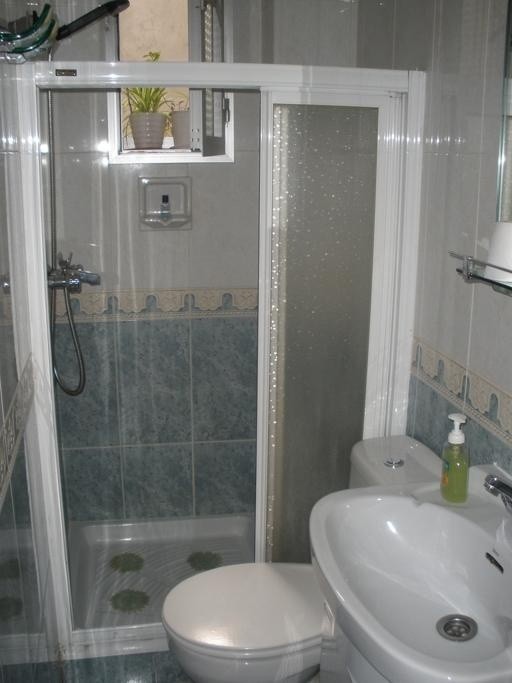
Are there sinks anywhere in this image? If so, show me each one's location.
[310,465,512,682]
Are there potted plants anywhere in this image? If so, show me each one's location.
[119,53,193,149]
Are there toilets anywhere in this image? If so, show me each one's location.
[161,435,448,683]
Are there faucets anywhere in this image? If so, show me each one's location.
[484,475,512,510]
